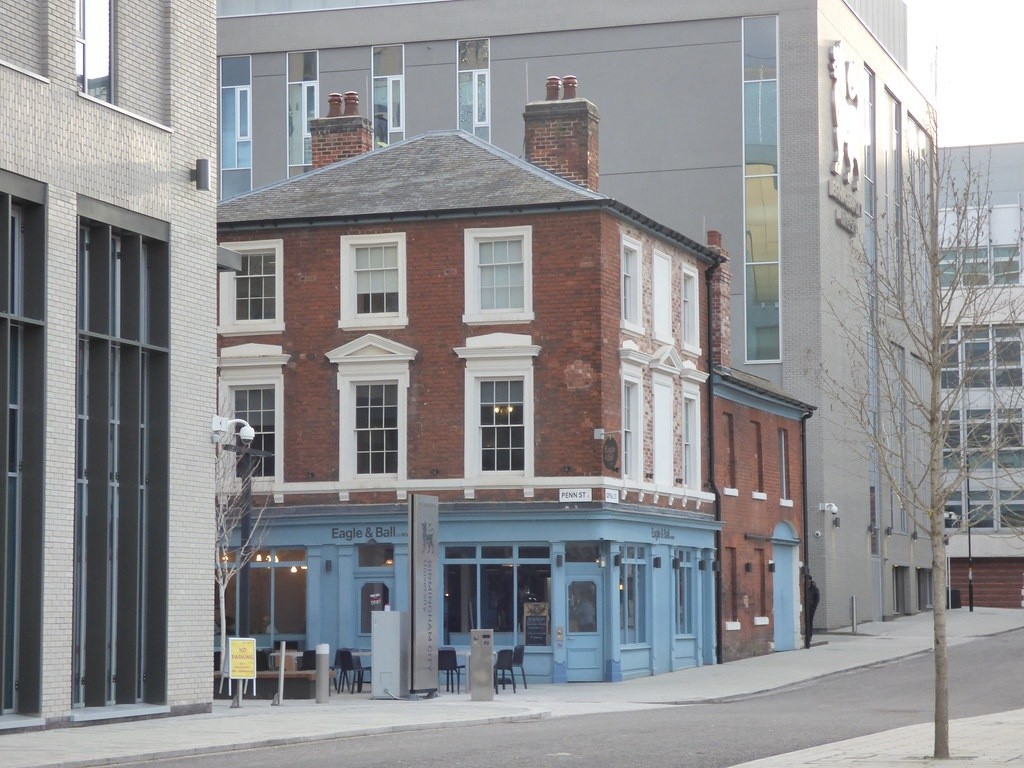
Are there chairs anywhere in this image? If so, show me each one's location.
[330,648,349,691]
[337,652,361,694]
[502,645,530,689]
[436,647,466,694]
[350,656,371,692]
[495,649,518,694]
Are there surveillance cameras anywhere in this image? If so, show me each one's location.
[831,506,839,514]
[240,425,255,445]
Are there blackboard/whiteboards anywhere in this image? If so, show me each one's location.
[525,615,547,646]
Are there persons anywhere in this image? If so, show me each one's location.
[808,574,822,642]
